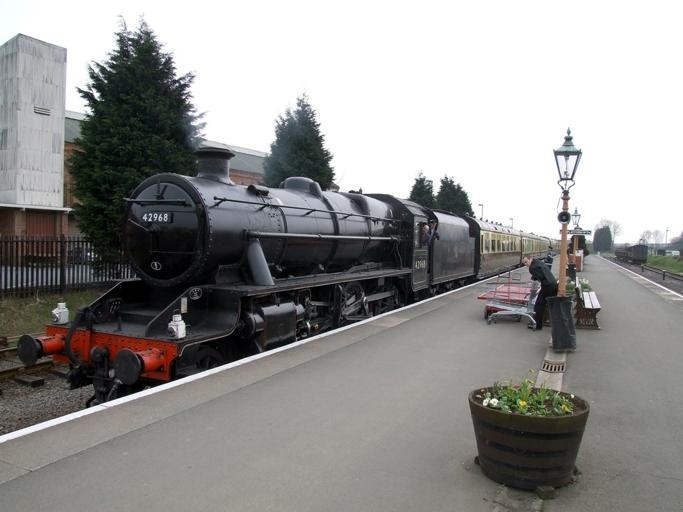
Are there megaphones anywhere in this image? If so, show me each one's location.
[557,211,571,224]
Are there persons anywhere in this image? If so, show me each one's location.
[522,254,558,330]
[539,252,553,271]
[421,224,431,247]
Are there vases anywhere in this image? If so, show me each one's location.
[468,387,590,491]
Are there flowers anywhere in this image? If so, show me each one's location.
[475,368,575,416]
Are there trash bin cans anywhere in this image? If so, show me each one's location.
[546,296,577,352]
[566,264,576,285]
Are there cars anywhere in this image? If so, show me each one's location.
[67,245,98,265]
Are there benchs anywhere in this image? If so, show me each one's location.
[574,276,601,329]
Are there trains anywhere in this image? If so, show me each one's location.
[15,141,560,406]
[615,243,649,266]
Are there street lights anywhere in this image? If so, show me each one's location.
[507,217,515,227]
[548,128,583,295]
[569,206,583,266]
[476,203,485,216]
[665,229,670,249]
